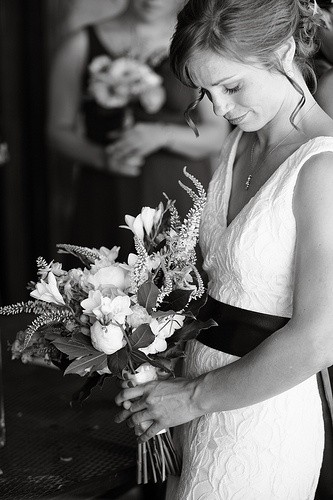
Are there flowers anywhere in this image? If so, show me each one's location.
[0,165,219,485]
[87,55,166,177]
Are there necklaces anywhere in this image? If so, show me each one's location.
[244,101,317,189]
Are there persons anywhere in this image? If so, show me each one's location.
[36,1,234,275]
[112,0,332,500]
